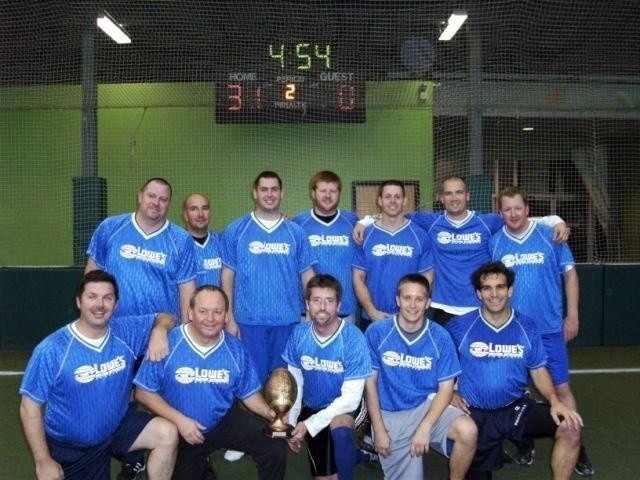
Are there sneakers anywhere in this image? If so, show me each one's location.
[117,458,146,480]
[572,444,595,476]
[358,433,382,468]
[513,445,536,466]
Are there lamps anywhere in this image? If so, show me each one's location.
[433,9,468,41]
[97,9,132,44]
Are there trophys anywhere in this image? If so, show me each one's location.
[262,366,298,441]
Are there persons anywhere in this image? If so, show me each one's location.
[179,191,226,293]
[285,170,364,332]
[486,184,597,478]
[440,260,586,480]
[361,273,480,479]
[126,283,303,480]
[345,178,437,330]
[214,170,319,462]
[350,175,572,331]
[11,268,181,479]
[279,273,379,479]
[78,177,199,480]
